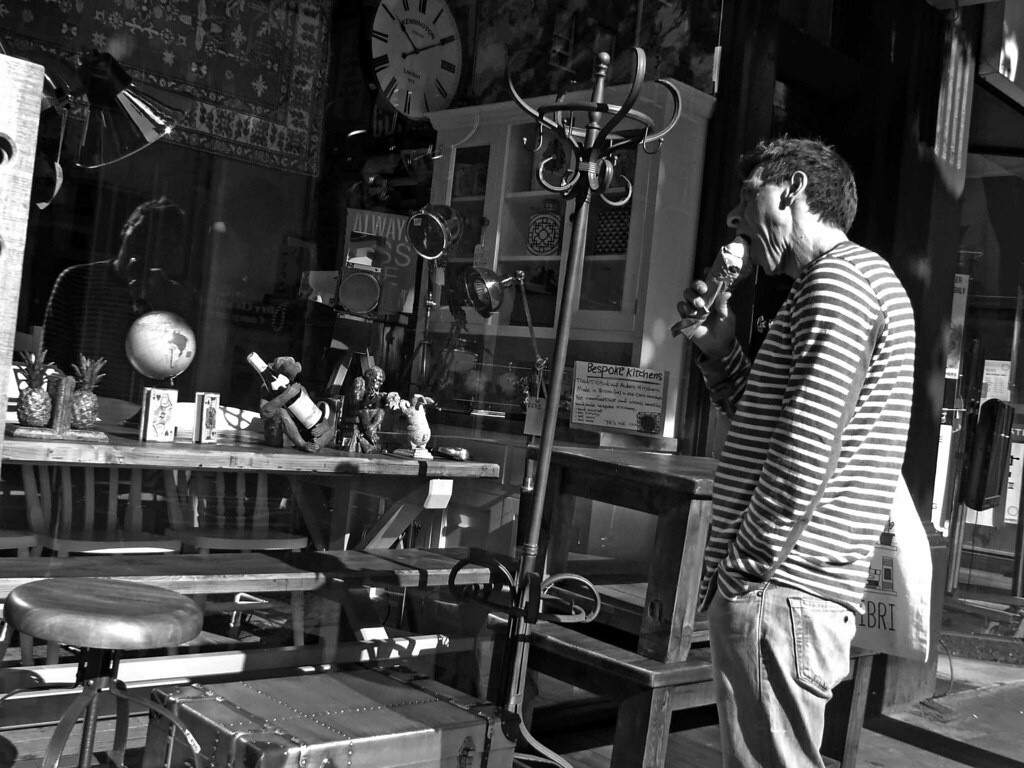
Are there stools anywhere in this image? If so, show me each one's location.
[1,576,205,768]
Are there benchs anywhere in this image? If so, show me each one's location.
[1,549,641,597]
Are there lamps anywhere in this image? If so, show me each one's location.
[405,200,547,420]
[41,45,183,169]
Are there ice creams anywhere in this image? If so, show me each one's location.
[710,236,752,297]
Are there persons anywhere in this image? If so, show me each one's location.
[355,366,388,443]
[38,196,188,407]
[677,137,916,768]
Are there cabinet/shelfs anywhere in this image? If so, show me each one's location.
[393,78,718,631]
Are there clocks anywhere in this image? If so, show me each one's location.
[361,1,466,129]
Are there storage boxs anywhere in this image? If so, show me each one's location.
[140,666,521,768]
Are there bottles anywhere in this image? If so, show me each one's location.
[246,351,324,441]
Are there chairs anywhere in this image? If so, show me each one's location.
[166,403,313,647]
[2,328,181,664]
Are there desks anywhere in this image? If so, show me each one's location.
[385,444,877,768]
[1,424,501,704]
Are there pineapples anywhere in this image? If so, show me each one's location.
[68,353,107,431]
[17,350,56,428]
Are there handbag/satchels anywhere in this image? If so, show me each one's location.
[850,471,933,663]
